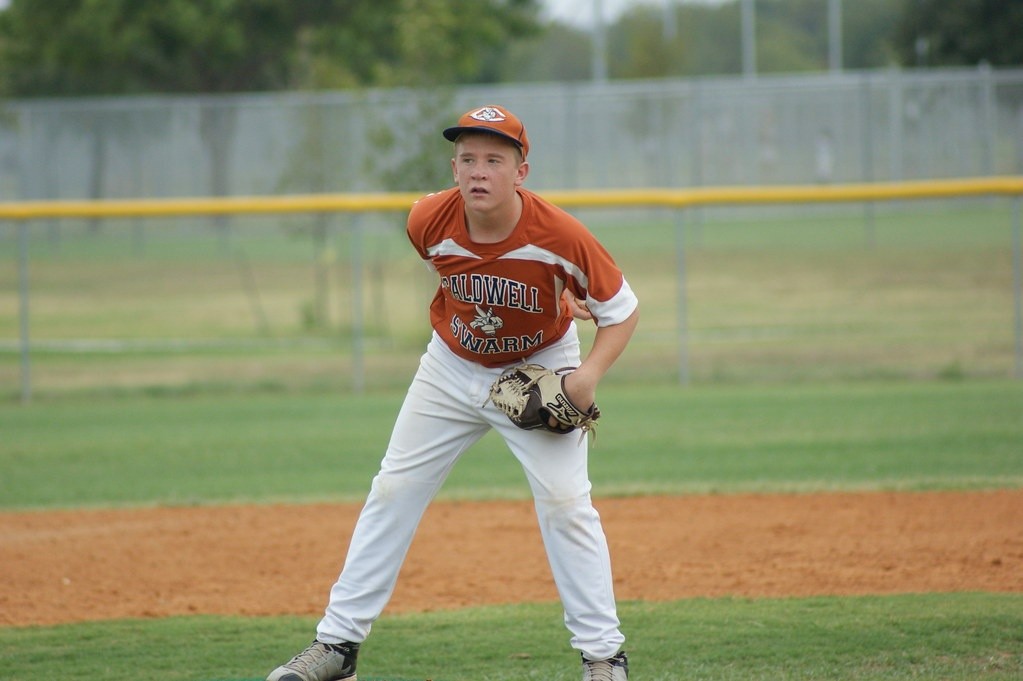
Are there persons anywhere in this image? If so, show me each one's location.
[264,106,640,680]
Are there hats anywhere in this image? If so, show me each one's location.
[442,105,529,162]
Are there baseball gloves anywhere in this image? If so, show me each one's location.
[489,364,601,436]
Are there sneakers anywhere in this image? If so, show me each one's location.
[265,638,361,681]
[581,649,628,681]
